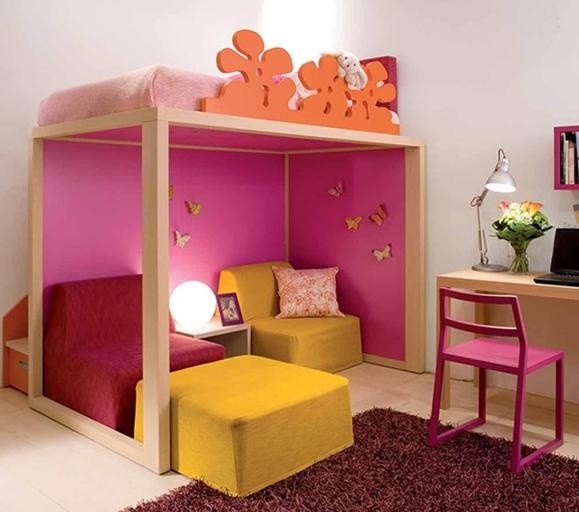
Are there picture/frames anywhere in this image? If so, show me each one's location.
[215,292,244,327]
[554,124,579,191]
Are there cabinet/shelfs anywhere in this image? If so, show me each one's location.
[5,337,28,397]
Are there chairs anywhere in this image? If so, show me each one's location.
[216,261,364,375]
[426,287,565,475]
[44,273,225,435]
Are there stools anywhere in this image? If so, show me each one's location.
[134,354,356,497]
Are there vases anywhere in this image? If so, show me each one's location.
[504,250,532,271]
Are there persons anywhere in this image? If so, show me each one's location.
[222,298,239,320]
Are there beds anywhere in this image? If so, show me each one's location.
[27,29,403,139]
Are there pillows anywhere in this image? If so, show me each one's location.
[269,263,344,318]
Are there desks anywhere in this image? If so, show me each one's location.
[436,268,579,411]
[175,315,251,355]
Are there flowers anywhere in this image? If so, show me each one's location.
[488,198,552,271]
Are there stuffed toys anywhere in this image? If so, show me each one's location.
[319,49,368,92]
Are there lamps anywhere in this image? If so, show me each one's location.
[470,146,517,272]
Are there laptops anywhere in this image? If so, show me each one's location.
[534,228,579,286]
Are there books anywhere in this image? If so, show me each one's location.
[560,128,579,184]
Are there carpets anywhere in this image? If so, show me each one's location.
[121,407,579,512]
